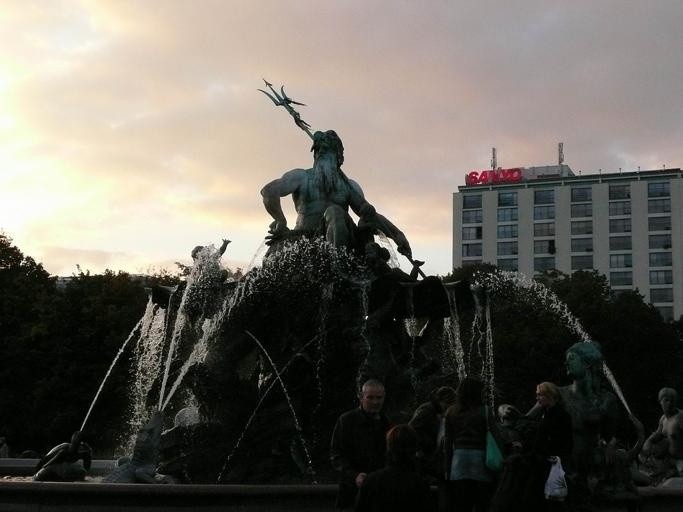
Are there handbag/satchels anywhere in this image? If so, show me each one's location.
[542,455,571,504]
[483,429,525,483]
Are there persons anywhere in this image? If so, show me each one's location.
[375,244,426,283]
[497,381,572,512]
[327,379,389,512]
[408,384,454,485]
[260,130,412,271]
[360,242,382,293]
[440,374,513,511]
[496,337,644,502]
[353,422,434,511]
[634,385,683,493]
